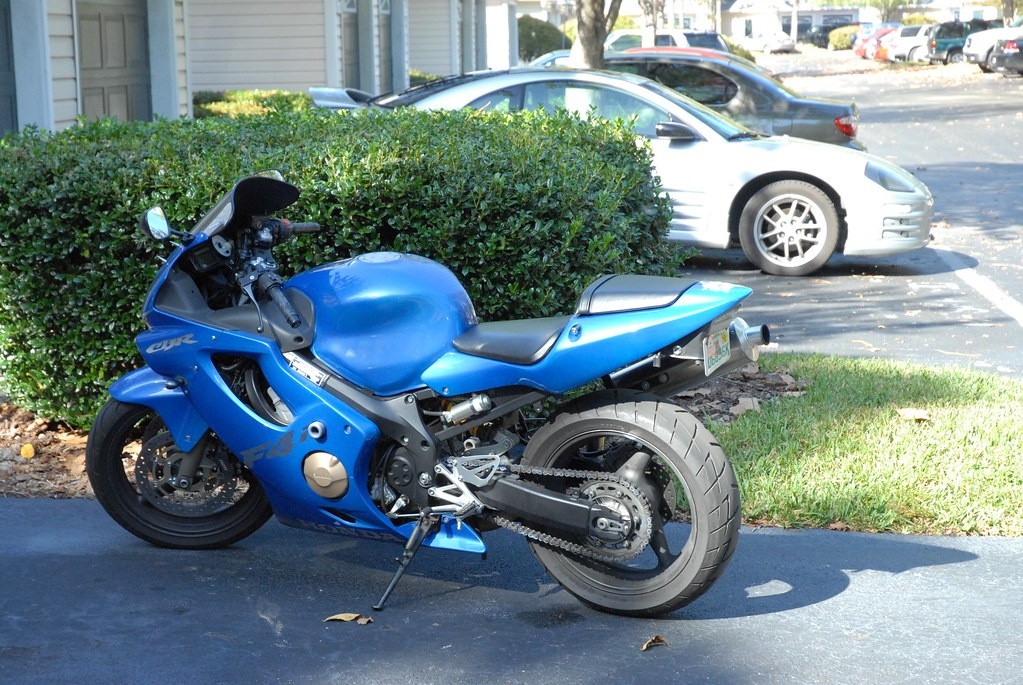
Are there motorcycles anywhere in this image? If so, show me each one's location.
[83,165,772,617]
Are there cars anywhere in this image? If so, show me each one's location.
[736,27,795,56]
[812,14,1023,74]
[525,27,870,154]
[305,66,937,277]
